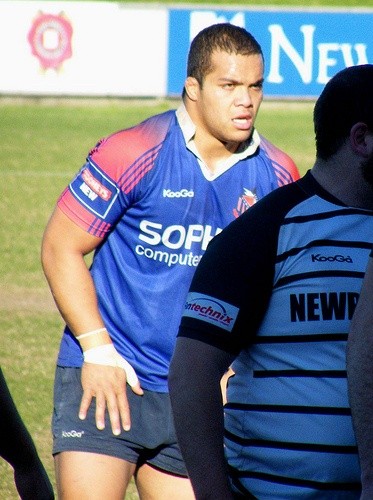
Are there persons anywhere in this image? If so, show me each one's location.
[0,370,54,500]
[344,251,373,500]
[169,64,373,500]
[40,24,302,500]
